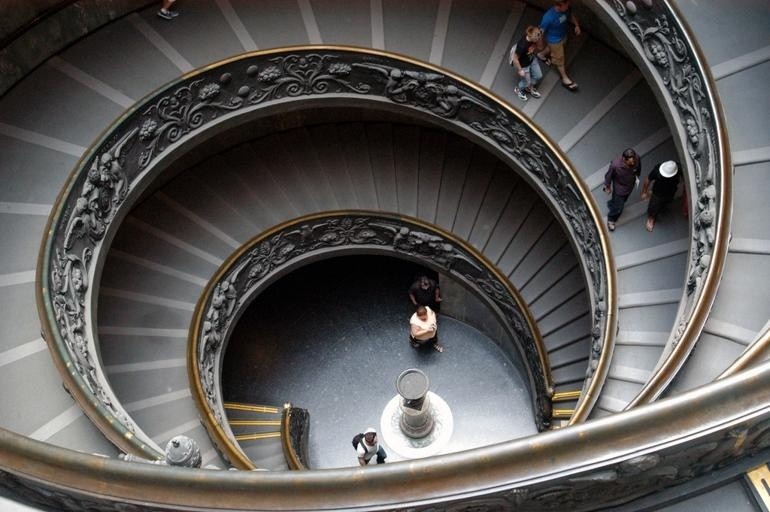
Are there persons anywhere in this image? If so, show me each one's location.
[601,147,641,232]
[507,23,544,101]
[537,1,582,93]
[409,305,443,352]
[407,274,443,311]
[641,159,683,232]
[155,0,181,21]
[351,426,387,465]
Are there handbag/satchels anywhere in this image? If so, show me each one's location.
[509,42,518,65]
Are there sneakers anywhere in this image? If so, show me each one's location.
[433,344,444,353]
[607,220,616,232]
[514,87,528,101]
[646,219,655,232]
[409,340,420,348]
[530,88,541,98]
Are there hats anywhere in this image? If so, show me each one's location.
[659,160,679,178]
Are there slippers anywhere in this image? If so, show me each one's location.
[562,79,578,90]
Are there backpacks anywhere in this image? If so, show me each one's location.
[352,433,364,451]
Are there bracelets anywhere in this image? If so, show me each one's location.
[644,191,647,193]
[574,25,580,27]
